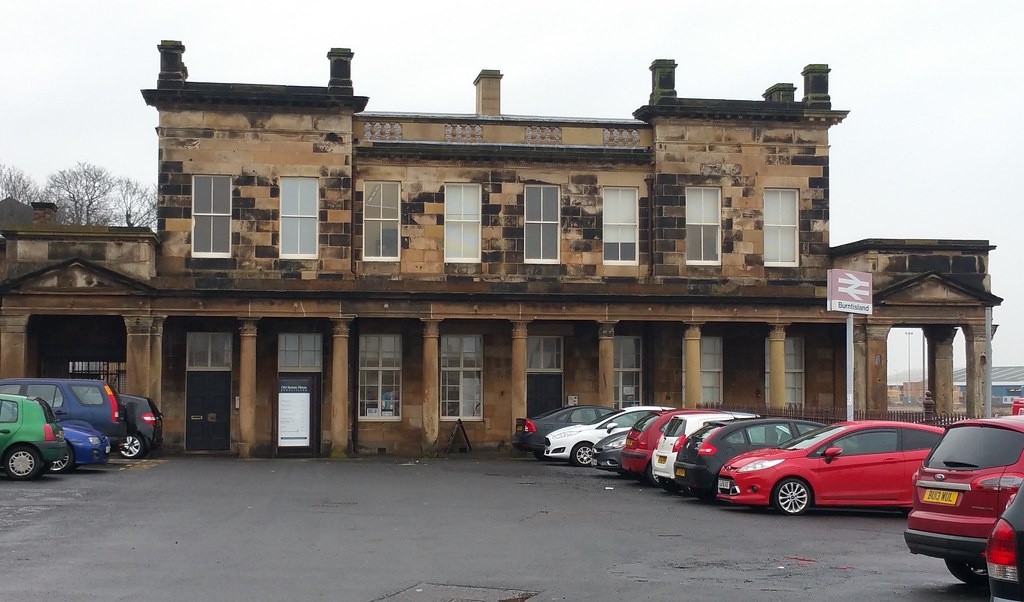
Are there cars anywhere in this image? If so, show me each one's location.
[716,417,947,516]
[512,404,619,463]
[619,408,719,488]
[590,427,631,477]
[0,394,68,481]
[117,393,163,460]
[650,411,795,500]
[542,402,672,467]
[58,422,111,472]
[905,414,1024,588]
[674,415,827,503]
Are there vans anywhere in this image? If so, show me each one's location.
[1,377,128,449]
